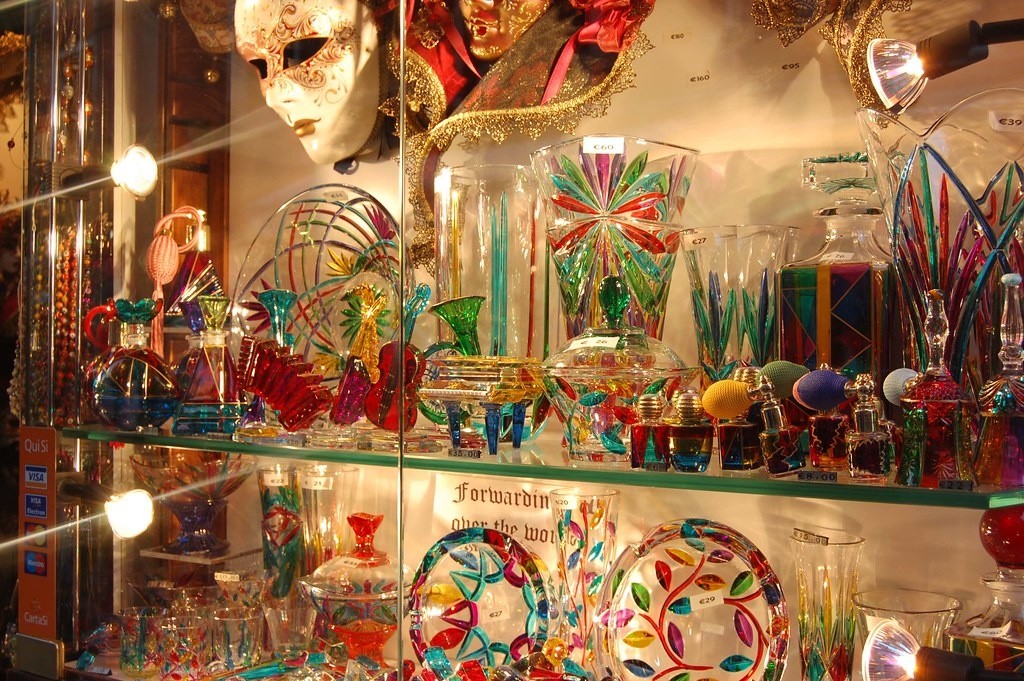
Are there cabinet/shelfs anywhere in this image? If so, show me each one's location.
[60,418,1024,681]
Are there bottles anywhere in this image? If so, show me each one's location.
[78,84,1024,680]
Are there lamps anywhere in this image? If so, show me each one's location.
[866,17,1024,114]
[57,475,154,542]
[59,144,159,201]
[861,619,1024,681]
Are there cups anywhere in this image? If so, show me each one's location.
[118,596,318,681]
